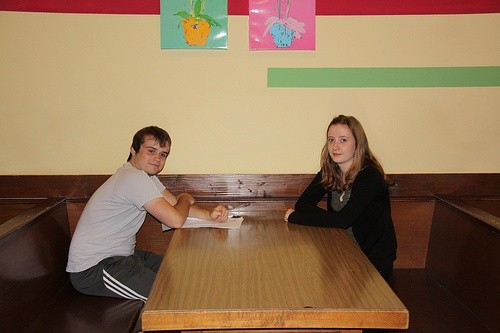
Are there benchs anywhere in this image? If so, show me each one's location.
[0,196,144,333]
[387,194,500,333]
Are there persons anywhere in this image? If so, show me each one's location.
[66,126,228,333]
[284,115,397,281]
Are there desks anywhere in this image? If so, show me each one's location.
[139,210,410,333]
